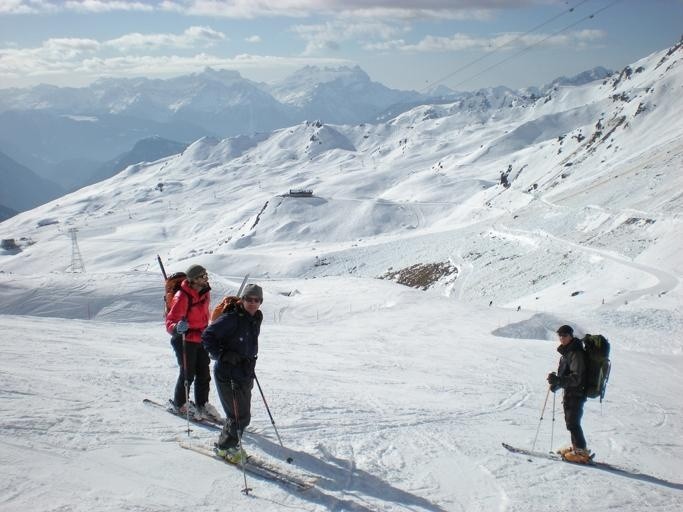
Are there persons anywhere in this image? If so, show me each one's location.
[197,283,262,464]
[546,324,589,463]
[163,263,219,424]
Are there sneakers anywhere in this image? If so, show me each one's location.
[213,445,246,465]
[167,400,201,421]
[565,450,592,464]
[197,402,225,425]
[559,445,591,454]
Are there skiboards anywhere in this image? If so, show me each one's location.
[501,442,638,479]
[141,398,261,439]
[175,435,318,491]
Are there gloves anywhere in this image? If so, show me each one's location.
[177,321,189,334]
[221,351,240,365]
[548,374,557,383]
[551,384,558,392]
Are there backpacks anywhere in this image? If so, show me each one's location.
[164,272,186,312]
[208,295,242,328]
[582,333,611,399]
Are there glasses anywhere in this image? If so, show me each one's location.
[197,273,208,279]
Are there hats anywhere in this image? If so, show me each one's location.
[186,265,205,281]
[242,284,263,300]
[556,325,573,334]
